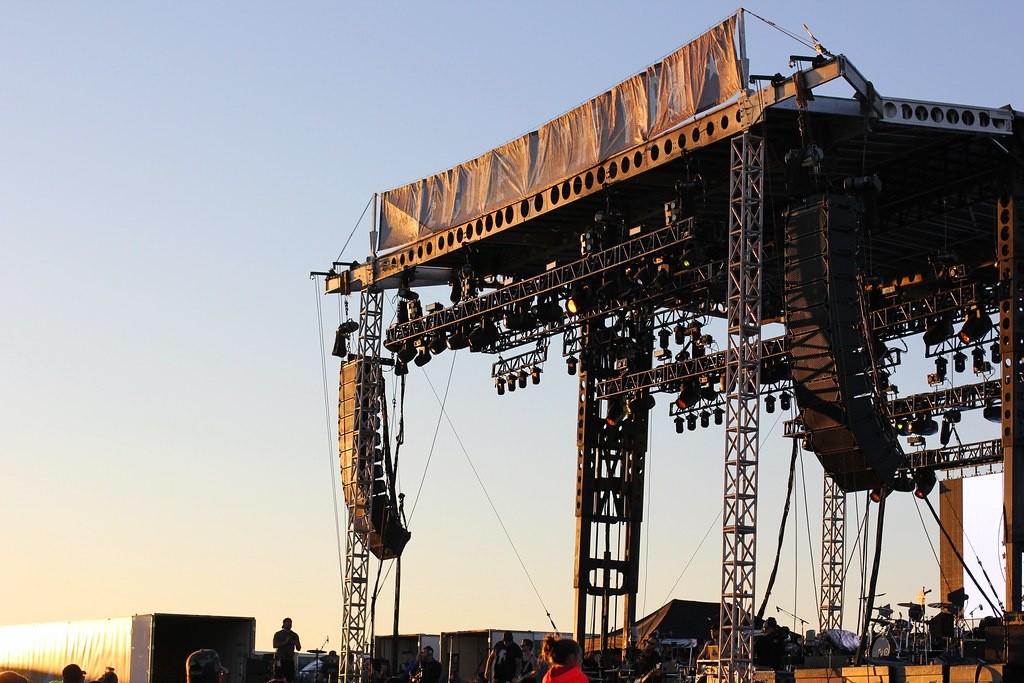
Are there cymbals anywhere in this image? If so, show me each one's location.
[872,607,894,613]
[897,603,921,608]
[870,618,892,627]
[927,602,953,609]
[307,650,327,653]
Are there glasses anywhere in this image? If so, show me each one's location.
[216,671,225,679]
[79,676,85,681]
[520,644,528,648]
[647,641,656,648]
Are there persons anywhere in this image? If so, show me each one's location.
[0,616,784,683]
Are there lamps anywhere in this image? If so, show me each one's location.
[381,158,1004,504]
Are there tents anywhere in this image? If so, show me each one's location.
[578,598,803,667]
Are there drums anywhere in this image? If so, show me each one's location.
[869,634,898,660]
[896,619,913,632]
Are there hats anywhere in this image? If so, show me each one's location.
[710,623,719,630]
[402,649,413,655]
[502,631,513,642]
[186,649,229,674]
[63,664,86,678]
[282,618,292,628]
